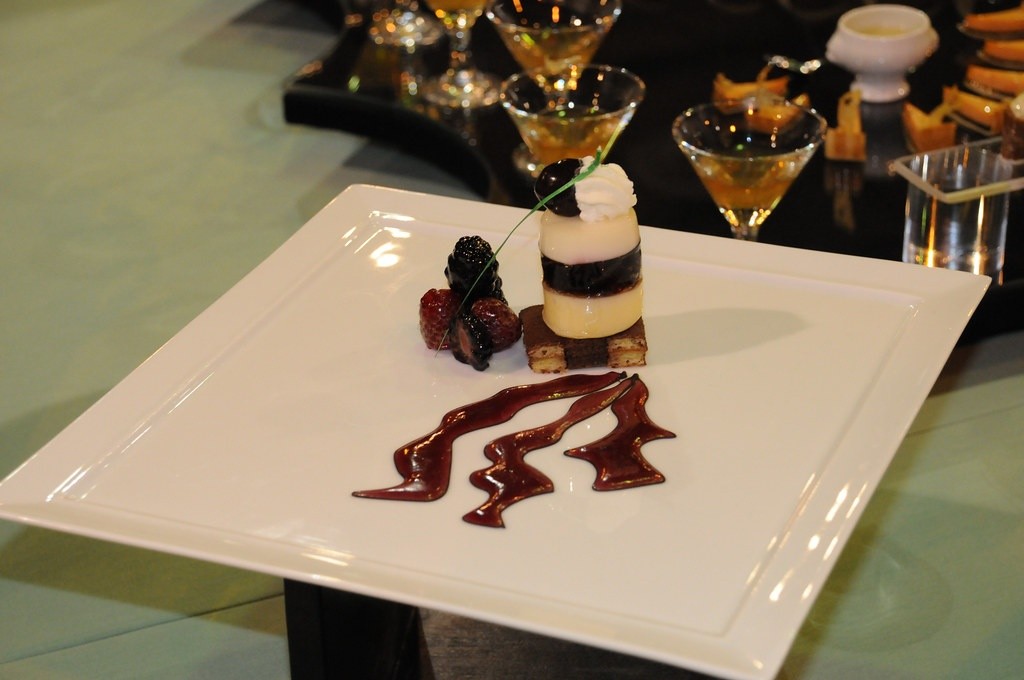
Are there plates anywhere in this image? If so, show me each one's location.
[0,181,990,680]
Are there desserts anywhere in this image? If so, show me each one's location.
[518,154,647,375]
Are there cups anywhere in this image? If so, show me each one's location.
[822,4,940,105]
[889,146,1020,277]
[672,96,829,243]
[364,0,646,167]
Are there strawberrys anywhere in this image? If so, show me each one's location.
[418,289,524,354]
